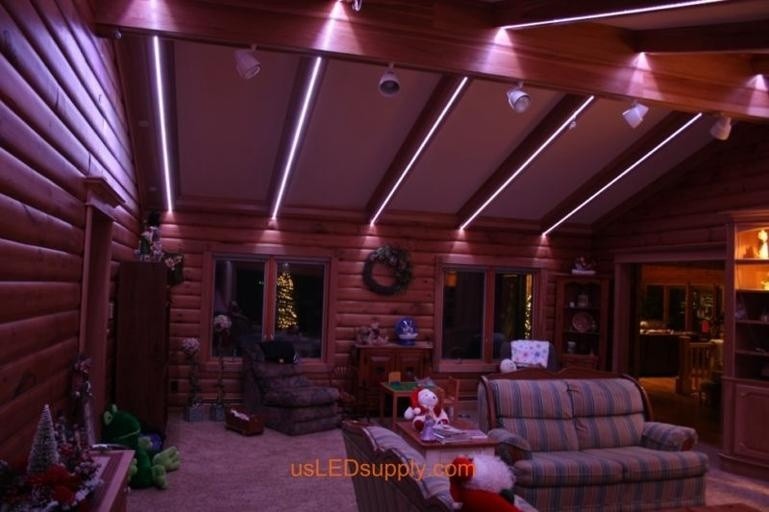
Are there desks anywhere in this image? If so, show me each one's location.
[59,448,138,512]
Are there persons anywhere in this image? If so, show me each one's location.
[403,385,451,430]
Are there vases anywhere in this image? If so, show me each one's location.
[184,404,225,426]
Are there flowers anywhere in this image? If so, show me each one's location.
[178,314,231,406]
[362,246,411,296]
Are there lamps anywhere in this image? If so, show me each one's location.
[232,48,736,142]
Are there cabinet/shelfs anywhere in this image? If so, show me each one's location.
[627,206,769,480]
[555,264,607,376]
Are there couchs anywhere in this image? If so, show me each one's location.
[340,336,709,511]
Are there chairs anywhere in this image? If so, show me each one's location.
[241,342,340,436]
